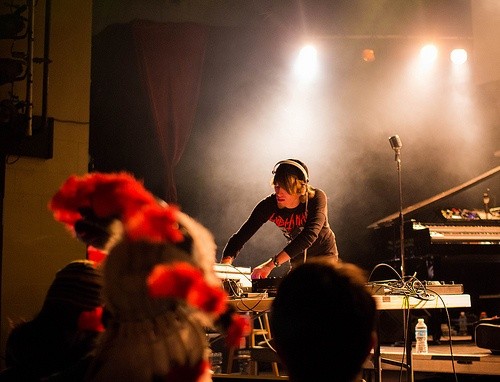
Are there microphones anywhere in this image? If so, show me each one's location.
[388,135,402,150]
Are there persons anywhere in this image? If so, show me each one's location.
[0,172,381,382]
[221,159,338,280]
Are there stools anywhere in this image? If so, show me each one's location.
[225,310,279,377]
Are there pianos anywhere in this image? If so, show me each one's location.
[364,165,500,345]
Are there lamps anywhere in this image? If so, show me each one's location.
[0,99,28,125]
[0,57,28,86]
[0,4,31,40]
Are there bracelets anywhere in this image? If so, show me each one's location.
[272,256,280,268]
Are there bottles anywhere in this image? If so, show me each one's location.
[459,312,467,335]
[480,311,487,318]
[414,318,427,353]
